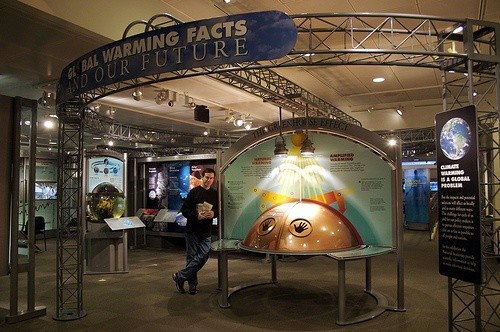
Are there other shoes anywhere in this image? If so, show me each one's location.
[189,285,197,294]
[172,273,186,294]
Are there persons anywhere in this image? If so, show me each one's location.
[172,168,218,295]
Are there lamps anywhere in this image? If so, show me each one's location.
[132,87,253,135]
[396,106,408,116]
[42,91,54,109]
[300,104,315,154]
[273,106,288,156]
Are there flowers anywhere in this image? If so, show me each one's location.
[92,196,115,223]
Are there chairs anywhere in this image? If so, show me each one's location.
[20,215,46,251]
